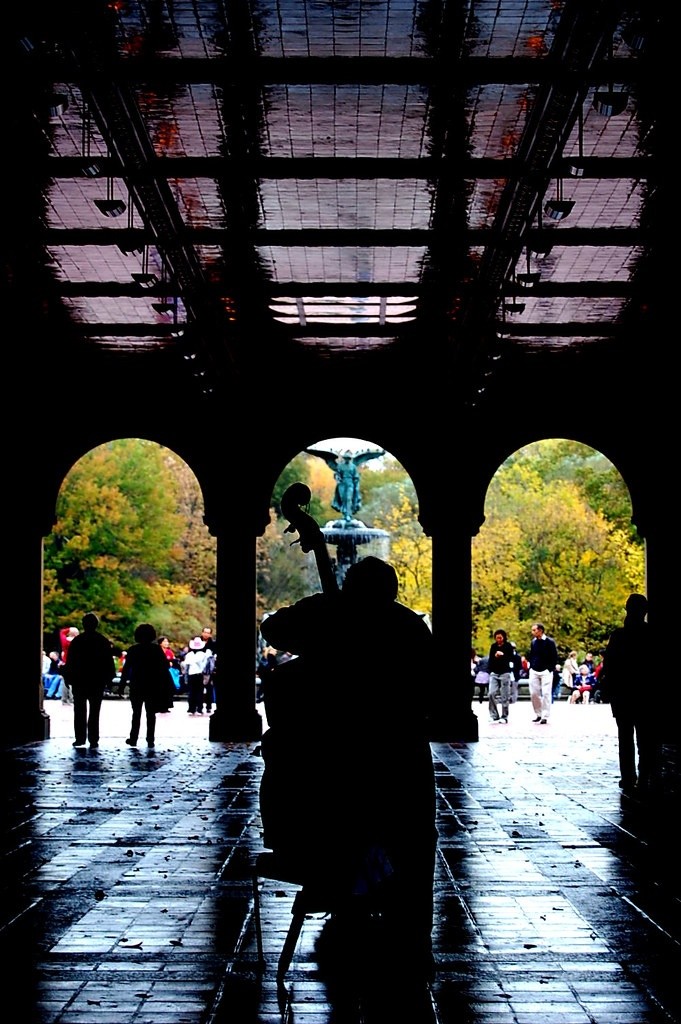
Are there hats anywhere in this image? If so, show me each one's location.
[189,637,206,650]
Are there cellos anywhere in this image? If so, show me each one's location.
[259,480,437,986]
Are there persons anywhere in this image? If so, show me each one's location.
[572,665,594,703]
[336,453,359,521]
[114,624,169,748]
[470,643,522,703]
[600,593,648,789]
[256,620,292,702]
[182,637,211,715]
[159,637,175,713]
[180,647,189,684]
[42,627,127,705]
[262,558,478,983]
[581,650,605,705]
[63,614,116,749]
[488,630,514,723]
[563,651,579,703]
[528,624,561,724]
[188,627,215,713]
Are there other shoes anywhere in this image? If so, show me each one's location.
[126,739,137,746]
[493,717,508,726]
[89,741,99,749]
[534,715,548,725]
[187,705,204,715]
[62,699,73,705]
[73,739,85,747]
[146,736,155,743]
